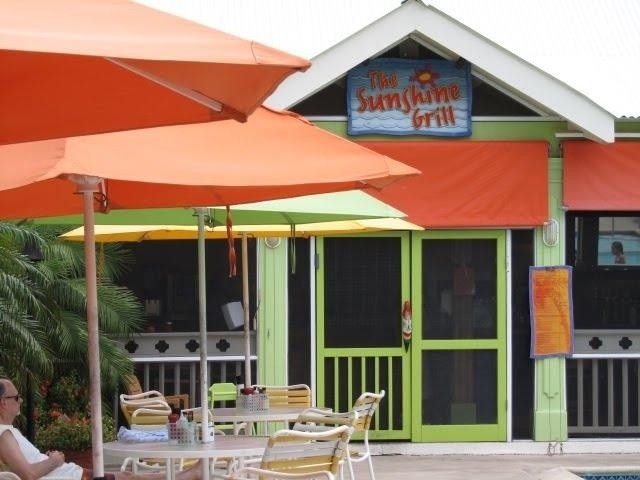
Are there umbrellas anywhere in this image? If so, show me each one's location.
[4,188,409,480]
[0,0,311,147]
[0,101,423,480]
[56,216,426,480]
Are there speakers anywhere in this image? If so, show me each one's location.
[220,301,244,331]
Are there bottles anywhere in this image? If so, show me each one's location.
[236,383,269,411]
[168,406,215,443]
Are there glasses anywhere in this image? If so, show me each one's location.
[3,392,20,402]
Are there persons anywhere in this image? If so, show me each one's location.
[0,378,213,480]
[611,240,626,264]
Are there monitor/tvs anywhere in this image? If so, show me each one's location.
[579,218,640,272]
